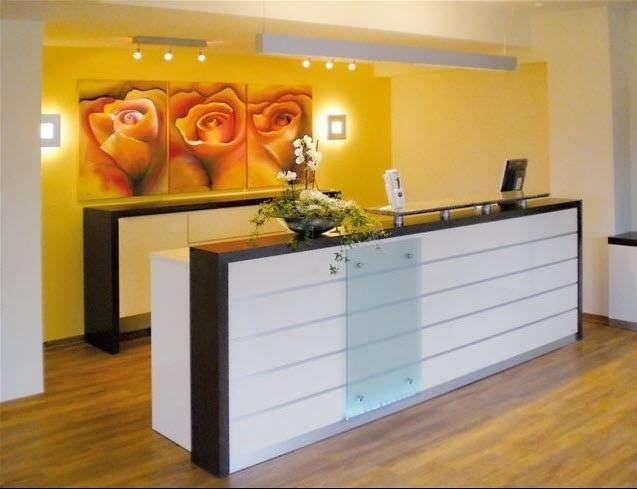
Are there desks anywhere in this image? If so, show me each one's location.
[608,231,637,331]
[149,197,583,479]
[83,189,340,355]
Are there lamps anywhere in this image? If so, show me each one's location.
[41,114,60,147]
[328,114,347,139]
[256,2,517,71]
[132,36,207,61]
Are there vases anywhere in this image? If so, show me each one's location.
[284,217,341,236]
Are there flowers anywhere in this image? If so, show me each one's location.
[252,136,391,274]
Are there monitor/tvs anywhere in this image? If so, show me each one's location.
[500,158,527,193]
